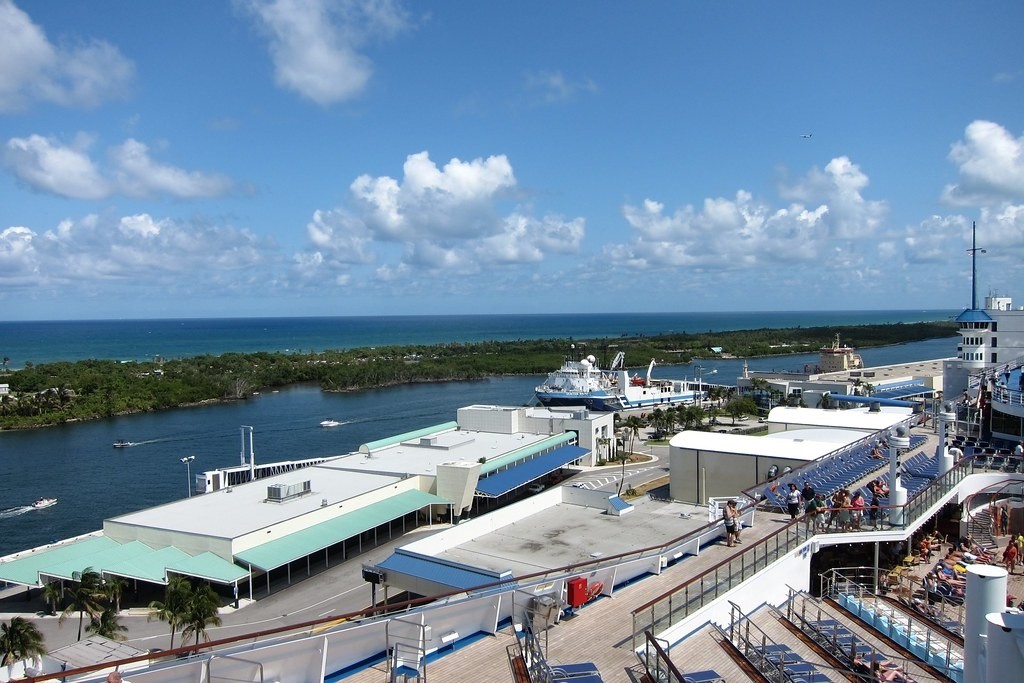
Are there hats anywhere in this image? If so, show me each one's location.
[937,566,942,570]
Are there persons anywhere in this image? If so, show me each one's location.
[853,653,904,671]
[723,500,738,547]
[947,443,964,471]
[883,438,889,448]
[870,444,884,459]
[872,661,915,683]
[784,479,891,533]
[845,508,1024,610]
[732,501,743,544]
[962,364,1024,413]
[1013,439,1024,472]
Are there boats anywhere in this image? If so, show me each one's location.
[32,496,58,508]
[535,351,737,412]
[112,440,134,448]
[319,418,338,427]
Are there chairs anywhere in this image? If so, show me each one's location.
[525,434,1024,683]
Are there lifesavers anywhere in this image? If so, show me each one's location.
[588,581,605,599]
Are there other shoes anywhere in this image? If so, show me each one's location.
[727,544,736,547]
[734,538,742,543]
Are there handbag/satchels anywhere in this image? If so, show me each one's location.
[1002,555,1007,563]
